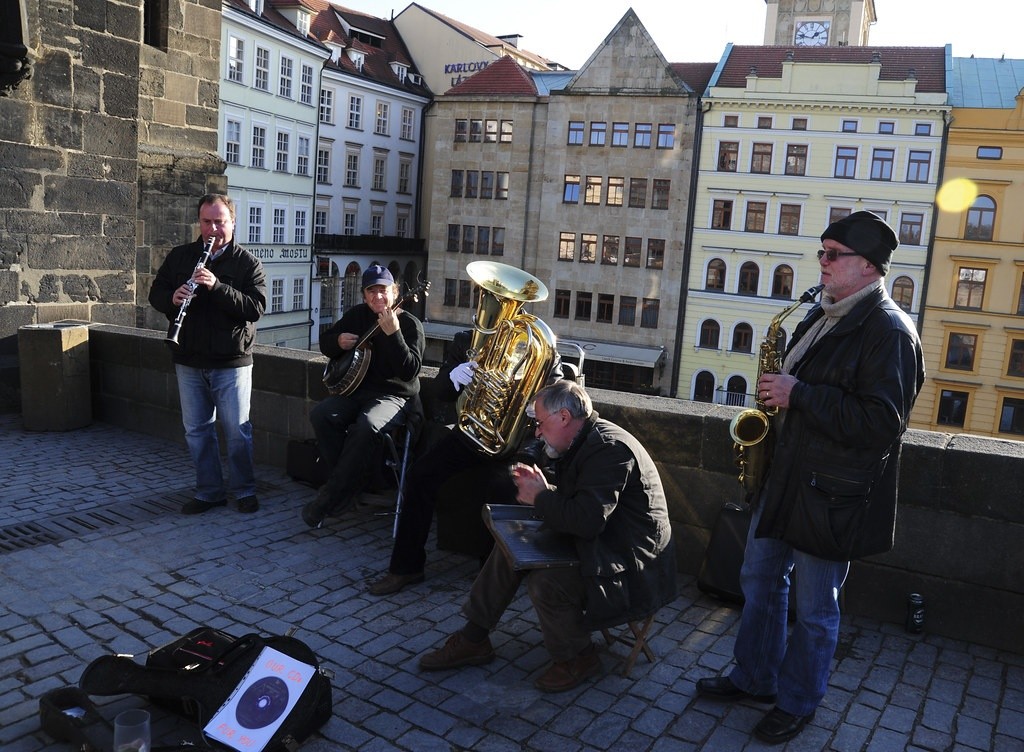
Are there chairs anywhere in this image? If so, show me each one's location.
[318,341,592,539]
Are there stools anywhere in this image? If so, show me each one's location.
[601,608,667,678]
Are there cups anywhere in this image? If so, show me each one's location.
[113,708,151,752]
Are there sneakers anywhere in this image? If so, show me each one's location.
[237,495,258,513]
[301,484,353,526]
[181,498,228,514]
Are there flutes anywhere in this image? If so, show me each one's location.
[164,234,217,346]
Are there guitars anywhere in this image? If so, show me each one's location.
[322,279,432,398]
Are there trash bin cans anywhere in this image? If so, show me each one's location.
[17,323,94,431]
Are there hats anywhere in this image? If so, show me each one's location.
[820,210,898,276]
[362,265,394,290]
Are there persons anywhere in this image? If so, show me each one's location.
[414,380,670,691]
[149,194,266,515]
[302,265,425,528]
[695,211,926,745]
[371,329,563,596]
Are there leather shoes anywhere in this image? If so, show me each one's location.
[368,570,424,594]
[420,630,496,670]
[696,676,777,704]
[755,706,816,744]
[535,643,602,692]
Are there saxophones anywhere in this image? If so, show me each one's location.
[729,283,826,494]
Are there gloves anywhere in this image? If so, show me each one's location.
[449,361,479,392]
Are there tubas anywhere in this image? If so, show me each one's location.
[457,259,557,458]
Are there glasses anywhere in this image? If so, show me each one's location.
[534,411,557,427]
[817,248,859,262]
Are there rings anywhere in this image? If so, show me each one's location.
[767,391,769,397]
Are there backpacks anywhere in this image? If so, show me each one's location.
[81,626,334,749]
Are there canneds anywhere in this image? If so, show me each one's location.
[907,594,924,634]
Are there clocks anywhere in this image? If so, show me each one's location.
[794,21,828,47]
